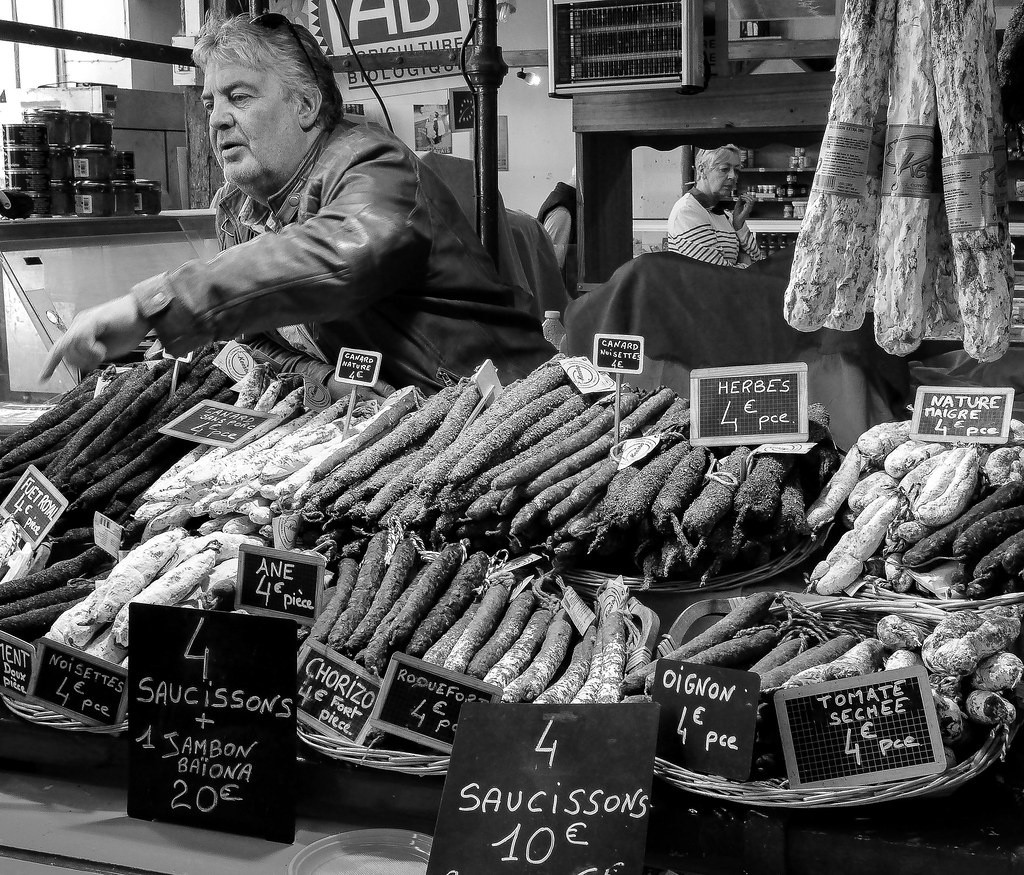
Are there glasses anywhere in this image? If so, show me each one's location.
[249,12,319,83]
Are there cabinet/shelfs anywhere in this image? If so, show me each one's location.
[721,166,817,218]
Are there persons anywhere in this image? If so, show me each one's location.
[667,144,761,270]
[40,12,564,399]
[537,163,578,303]
[426,111,446,146]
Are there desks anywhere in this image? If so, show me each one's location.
[0,211,222,404]
[0,764,437,875]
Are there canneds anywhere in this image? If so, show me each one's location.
[2,108,162,218]
[747,173,796,197]
[788,147,811,168]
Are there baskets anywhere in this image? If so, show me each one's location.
[0,513,1024,843]
